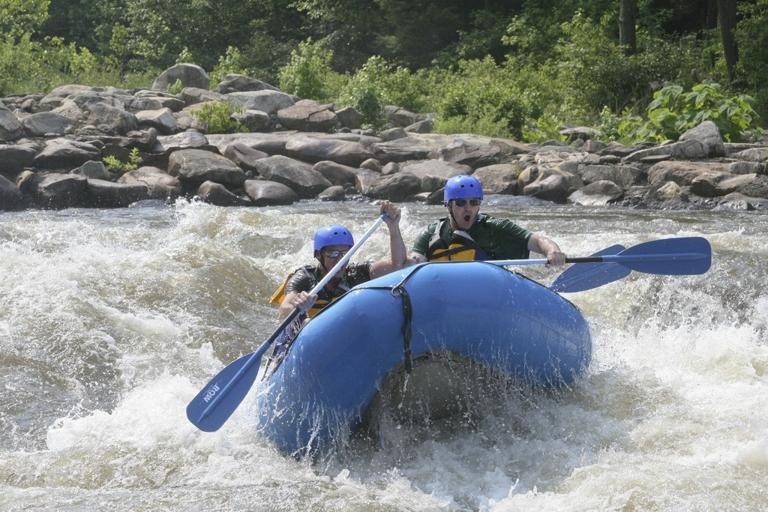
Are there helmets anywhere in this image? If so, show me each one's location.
[312,224,355,258]
[443,174,483,206]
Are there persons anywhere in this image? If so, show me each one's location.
[410,174,565,267]
[279,200,407,320]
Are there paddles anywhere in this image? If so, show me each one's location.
[480,237,712,293]
[185,208,391,433]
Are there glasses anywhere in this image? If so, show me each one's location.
[451,198,481,207]
[322,249,349,259]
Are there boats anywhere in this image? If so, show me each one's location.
[258,261,592,463]
